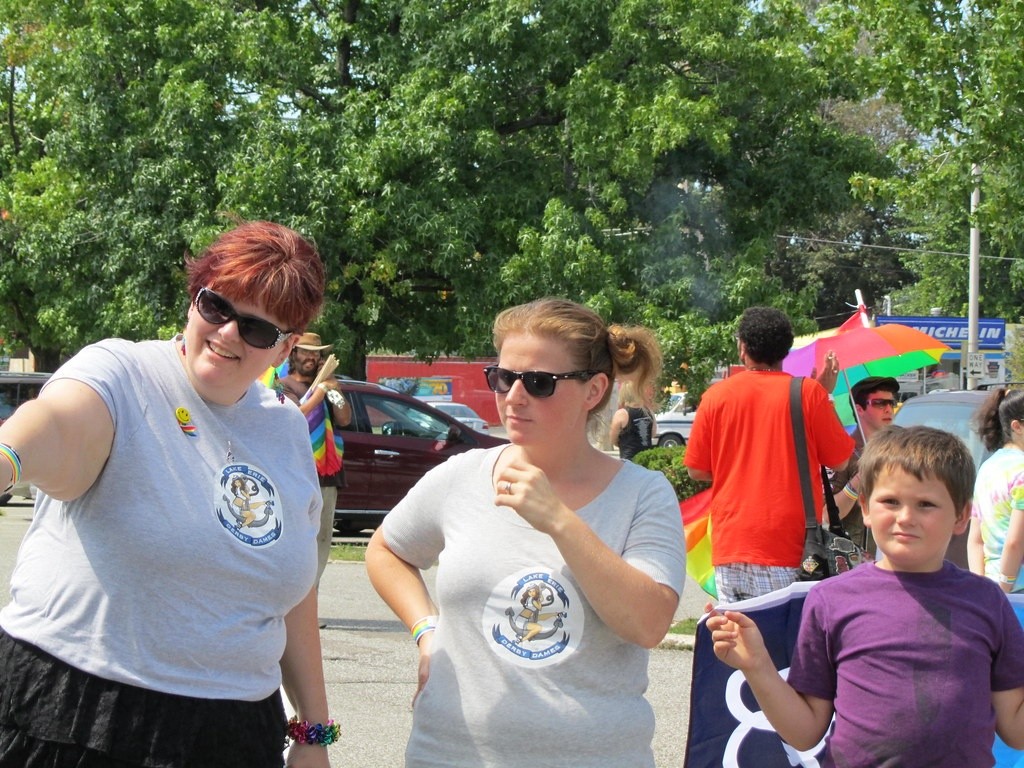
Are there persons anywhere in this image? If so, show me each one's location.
[610,381,657,460]
[684,306,856,608]
[0,220,330,768]
[822,376,899,557]
[705,426,1024,768]
[968,387,1024,596]
[273,334,351,628]
[362,297,686,768]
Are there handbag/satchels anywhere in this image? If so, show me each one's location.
[796,519,861,582]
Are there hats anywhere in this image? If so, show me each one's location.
[296,333,332,351]
[849,377,901,414]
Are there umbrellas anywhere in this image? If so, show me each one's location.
[782,325,954,444]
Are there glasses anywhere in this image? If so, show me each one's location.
[482,364,594,400]
[195,285,293,349]
[859,399,898,409]
[298,349,320,356]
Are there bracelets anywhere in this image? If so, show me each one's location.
[842,485,858,500]
[287,717,341,745]
[0,444,22,485]
[410,616,437,644]
[999,573,1016,584]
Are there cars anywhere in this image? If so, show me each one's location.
[892,389,998,473]
[407,400,491,436]
[655,391,699,450]
[331,373,511,535]
[0,370,54,507]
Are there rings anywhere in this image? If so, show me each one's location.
[832,371,837,373]
[505,482,512,494]
